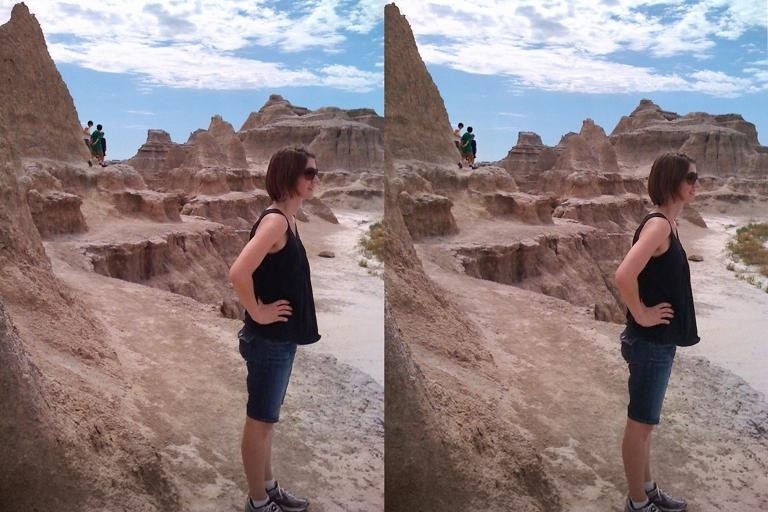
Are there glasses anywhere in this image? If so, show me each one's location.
[686,172,698,185]
[302,168,318,181]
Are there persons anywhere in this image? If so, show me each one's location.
[97,132,106,161]
[460,127,477,169]
[227,146,322,511]
[469,133,476,160]
[89,124,108,167]
[453,123,464,169]
[611,152,702,511]
[82,121,93,167]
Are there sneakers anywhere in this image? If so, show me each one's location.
[245,481,309,512]
[625,482,688,512]
